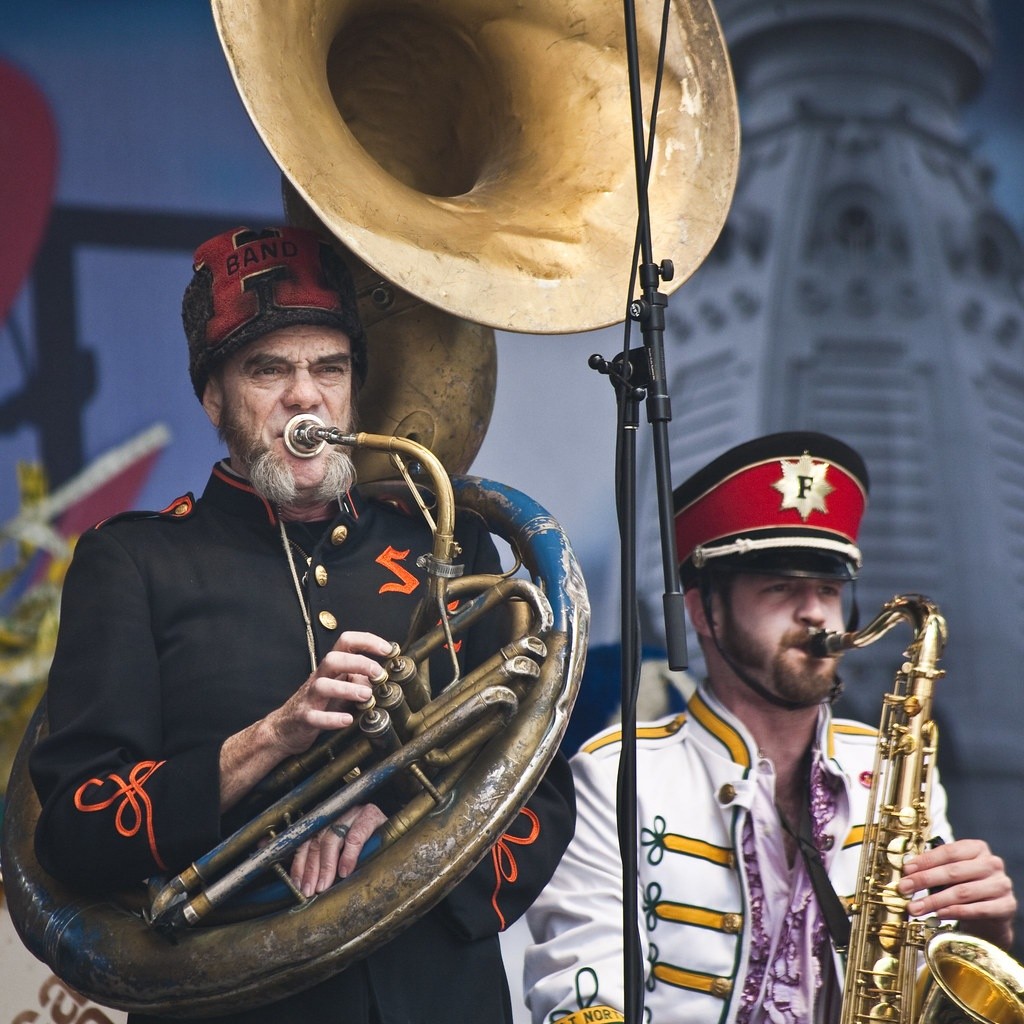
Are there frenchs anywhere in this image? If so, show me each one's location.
[1,1,741,1019]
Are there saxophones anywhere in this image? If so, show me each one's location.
[802,593,1024,1024]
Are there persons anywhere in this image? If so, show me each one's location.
[522,428,1021,1022]
[29,231,580,1024]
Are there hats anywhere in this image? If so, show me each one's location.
[179,227,366,408]
[670,430,869,595]
